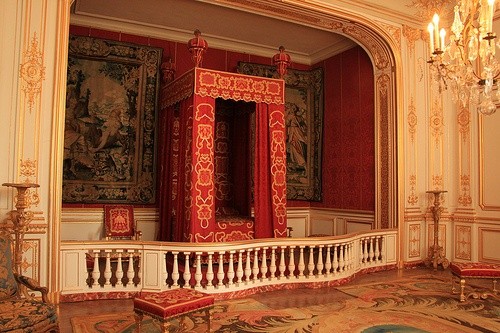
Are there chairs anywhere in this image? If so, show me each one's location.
[0,227,60,333]
[104,204,142,241]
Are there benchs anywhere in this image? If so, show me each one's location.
[134,289,214,333]
[449,261,500,302]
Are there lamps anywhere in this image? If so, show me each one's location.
[427,0,500,115]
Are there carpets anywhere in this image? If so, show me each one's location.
[71,274,500,333]
